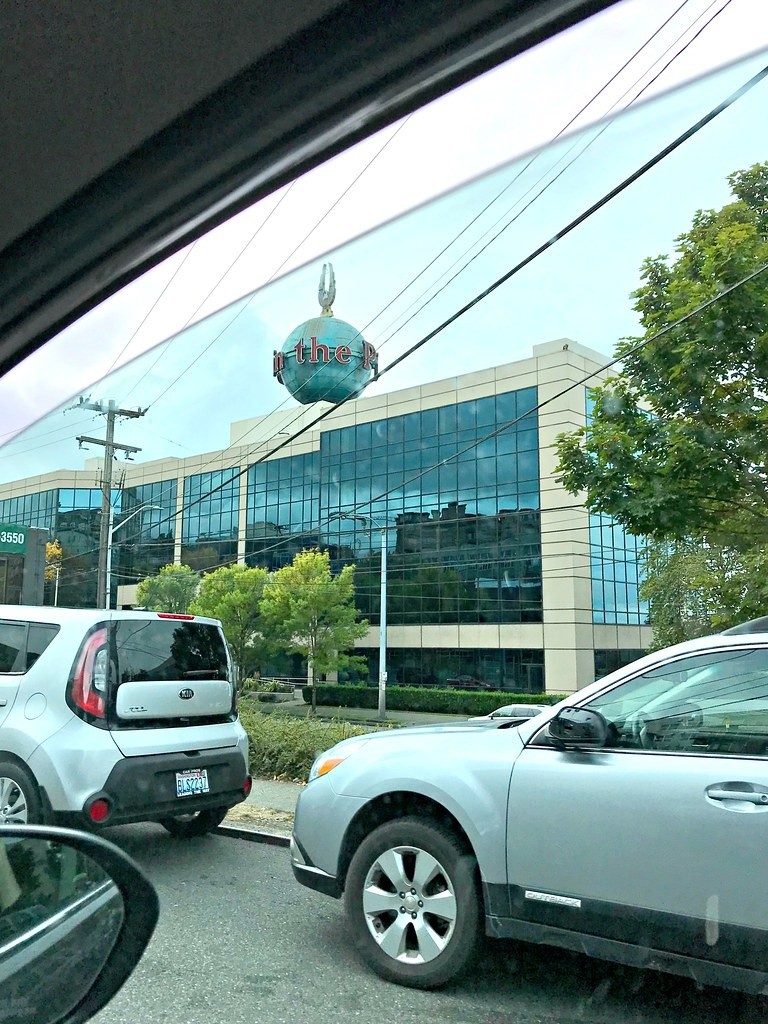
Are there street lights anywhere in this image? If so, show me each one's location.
[104,506,164,609]
[329,510,389,723]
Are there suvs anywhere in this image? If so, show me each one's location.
[289,615,768,1008]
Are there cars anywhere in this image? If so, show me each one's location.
[0,602,254,839]
[446,677,498,692]
[1,821,160,1024]
